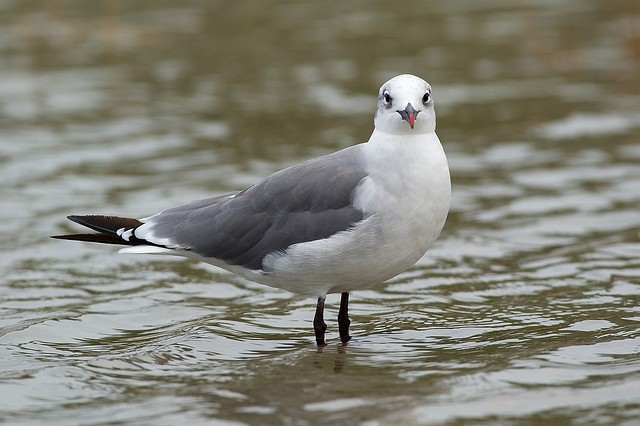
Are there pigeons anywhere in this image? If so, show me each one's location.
[49,75,451,347]
